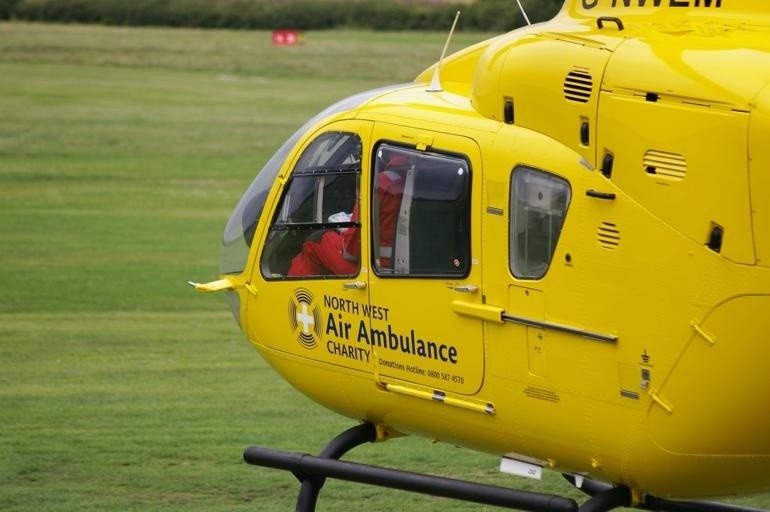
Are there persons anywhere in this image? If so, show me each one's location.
[287,152,409,275]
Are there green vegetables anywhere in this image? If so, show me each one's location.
[188,0,770,512]
